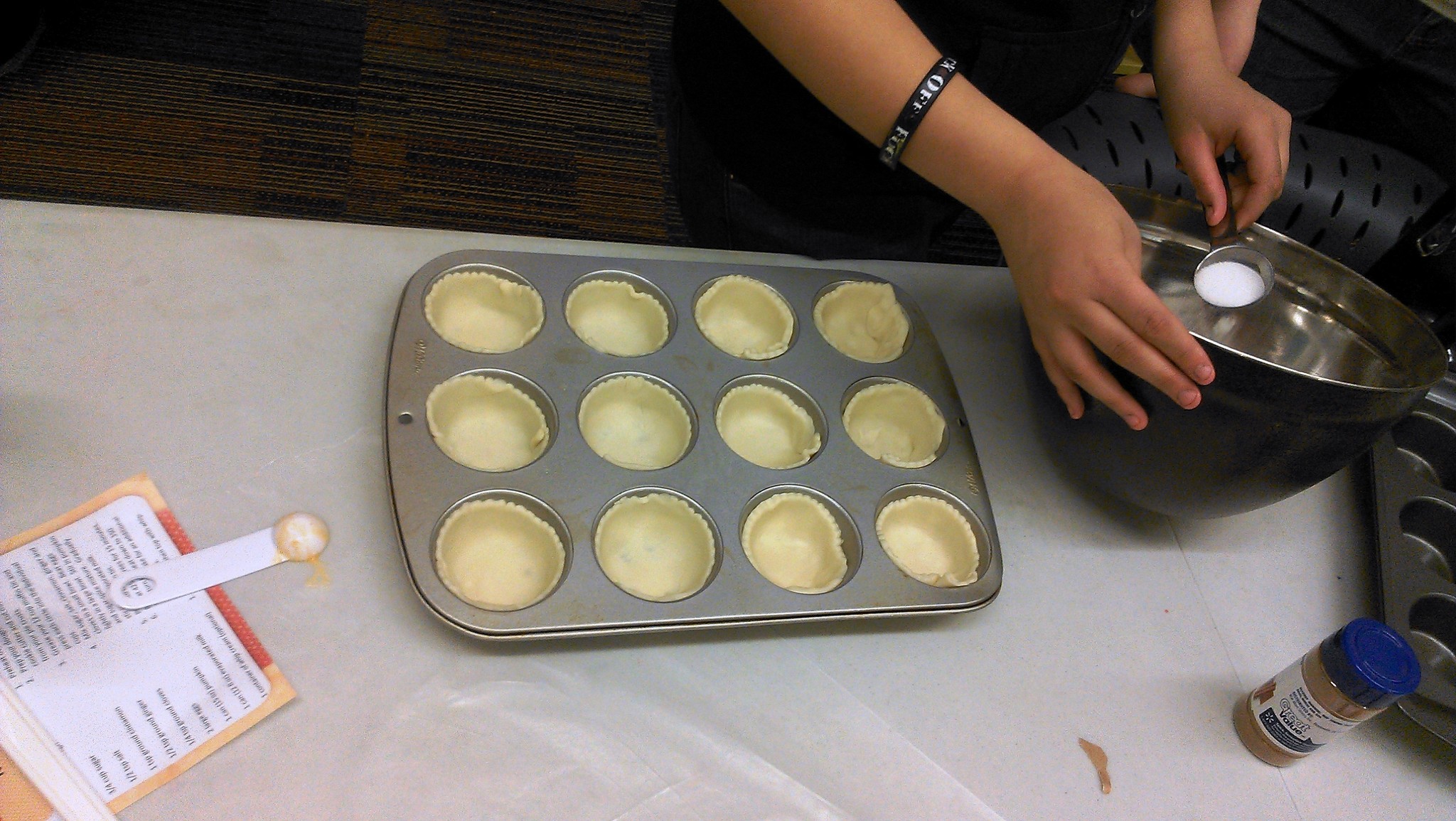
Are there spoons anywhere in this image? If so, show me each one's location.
[1195,152,1277,311]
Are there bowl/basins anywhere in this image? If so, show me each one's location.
[1041,184,1448,519]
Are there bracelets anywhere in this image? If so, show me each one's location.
[878,56,960,170]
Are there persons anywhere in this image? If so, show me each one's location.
[672,0,1291,430]
[1117,0,1456,188]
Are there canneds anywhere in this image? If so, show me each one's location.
[1232,616,1421,768]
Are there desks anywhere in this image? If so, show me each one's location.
[0,198,1456,821]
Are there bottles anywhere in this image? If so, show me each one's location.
[1232,616,1421,768]
